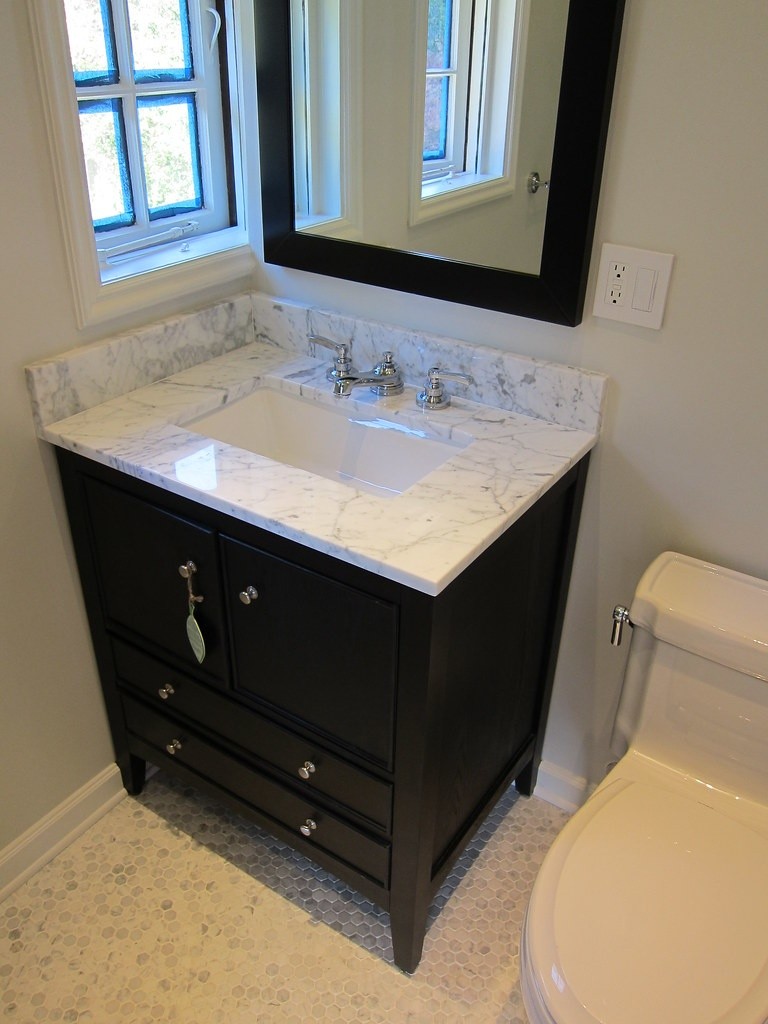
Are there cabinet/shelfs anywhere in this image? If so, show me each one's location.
[55,451,595,975]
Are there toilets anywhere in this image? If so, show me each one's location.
[517,548,768,1024]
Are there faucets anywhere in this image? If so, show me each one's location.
[331,351,404,401]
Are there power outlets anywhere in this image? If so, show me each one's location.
[589,243,672,329]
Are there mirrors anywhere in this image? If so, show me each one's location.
[250,0,625,328]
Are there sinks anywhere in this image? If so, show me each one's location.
[176,385,470,499]
